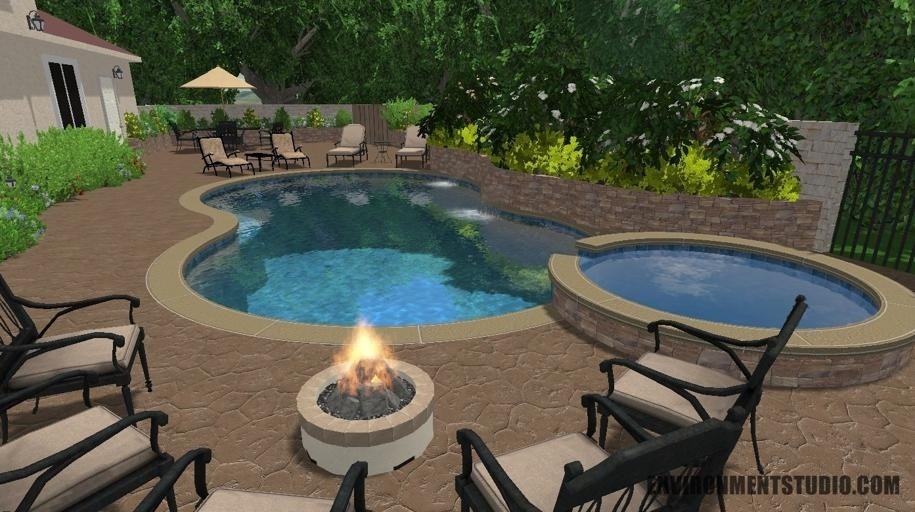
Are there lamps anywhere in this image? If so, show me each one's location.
[26,9,45,32]
